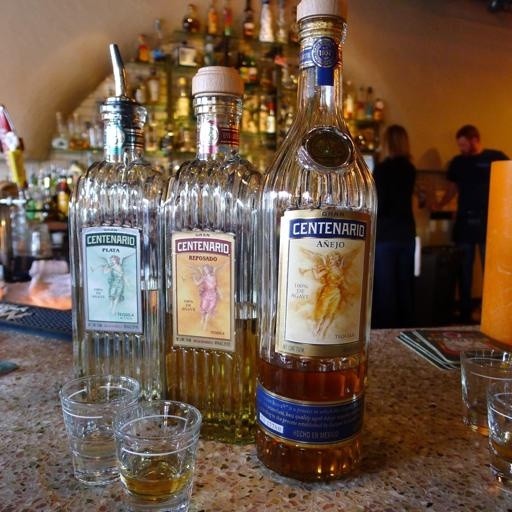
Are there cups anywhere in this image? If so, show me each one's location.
[458,347,511,437]
[485,379,512,487]
[111,398,203,512]
[59,373,140,486]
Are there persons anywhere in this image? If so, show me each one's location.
[438,124,510,312]
[371,124,415,328]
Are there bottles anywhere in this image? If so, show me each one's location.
[1,106,83,261]
[67,43,169,413]
[159,63,263,450]
[56,6,384,151]
[253,0,378,482]
